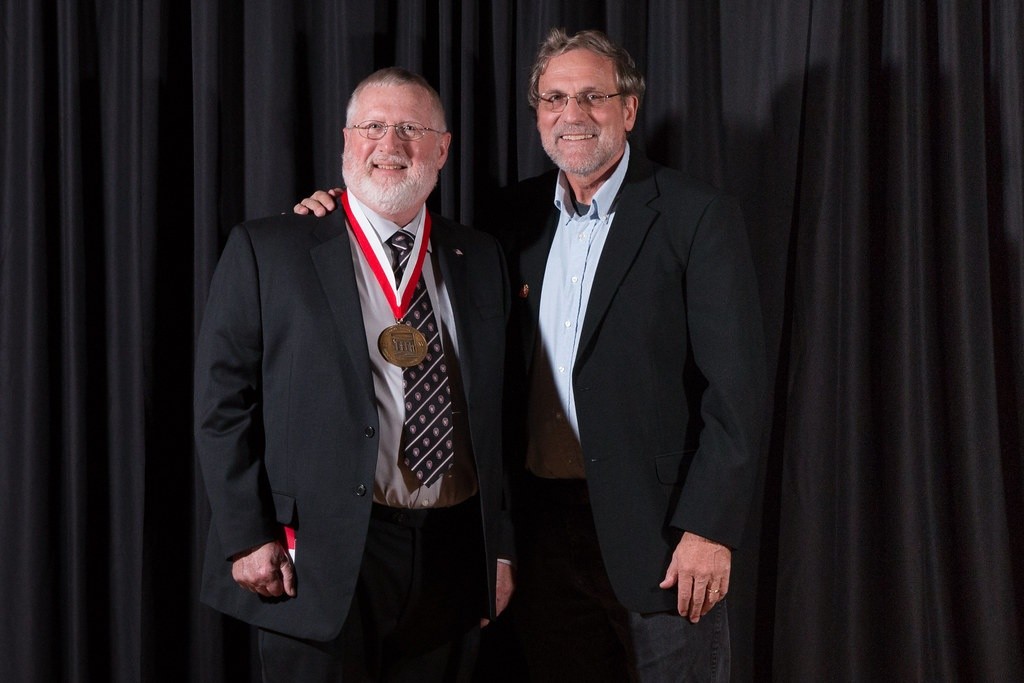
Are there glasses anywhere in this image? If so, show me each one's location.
[347,119,443,141]
[537,92,622,114]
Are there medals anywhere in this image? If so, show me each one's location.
[379,319,427,367]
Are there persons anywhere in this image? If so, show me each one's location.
[195,66,512,683]
[289,25,768,683]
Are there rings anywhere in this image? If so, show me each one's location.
[707,589,720,594]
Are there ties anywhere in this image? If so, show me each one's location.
[384,230,456,488]
